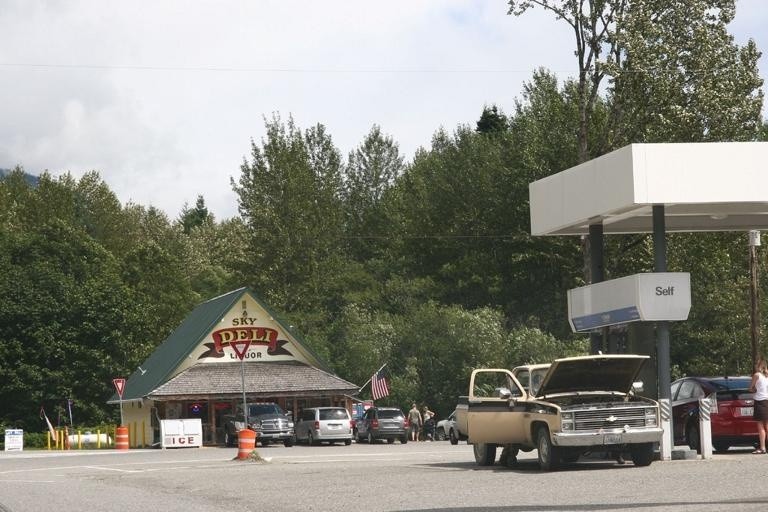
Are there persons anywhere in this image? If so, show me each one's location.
[371,365,389,401]
[748,358,768,455]
[422,405,435,442]
[406,403,422,441]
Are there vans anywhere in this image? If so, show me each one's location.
[294,407,353,446]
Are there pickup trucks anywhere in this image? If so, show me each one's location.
[456,352,662,470]
[223,401,295,446]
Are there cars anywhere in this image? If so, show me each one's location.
[435,408,468,444]
[672,375,766,454]
[354,406,408,445]
